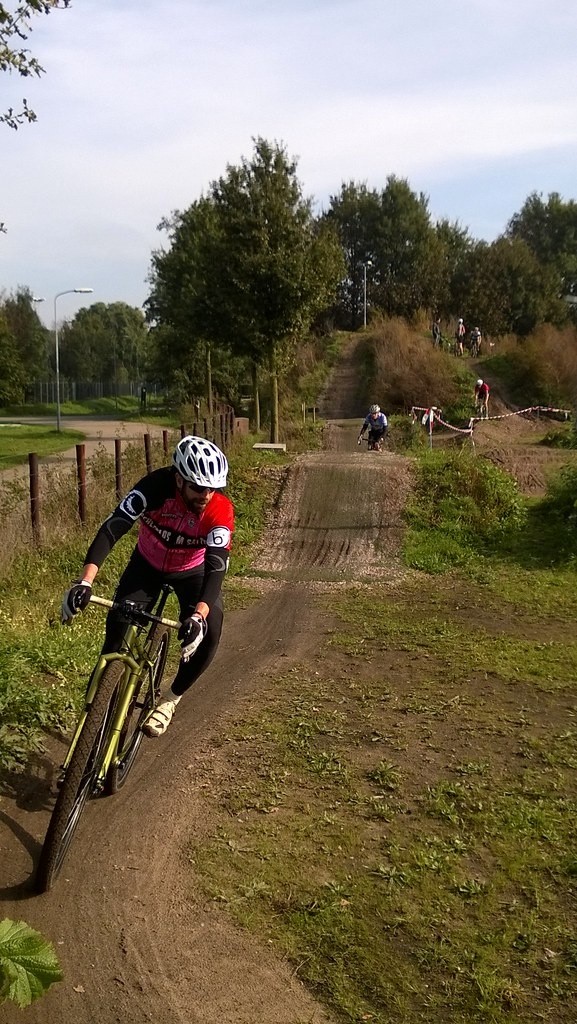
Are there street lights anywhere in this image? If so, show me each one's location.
[54,288,93,430]
[363,261,372,330]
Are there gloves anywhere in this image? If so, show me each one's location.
[358,435,362,444]
[380,438,383,442]
[62,580,92,626]
[178,613,208,663]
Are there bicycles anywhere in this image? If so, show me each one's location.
[451,336,461,357]
[35,583,194,892]
[470,341,480,358]
[474,398,486,421]
[358,437,382,451]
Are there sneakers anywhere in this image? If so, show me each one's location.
[56,772,66,788]
[142,687,182,738]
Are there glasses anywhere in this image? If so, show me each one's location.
[184,483,217,493]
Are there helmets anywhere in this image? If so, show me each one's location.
[458,318,463,323]
[476,380,483,386]
[173,435,228,488]
[369,404,380,413]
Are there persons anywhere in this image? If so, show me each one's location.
[357,404,388,452]
[474,379,490,418]
[433,316,482,357]
[57,434,238,792]
